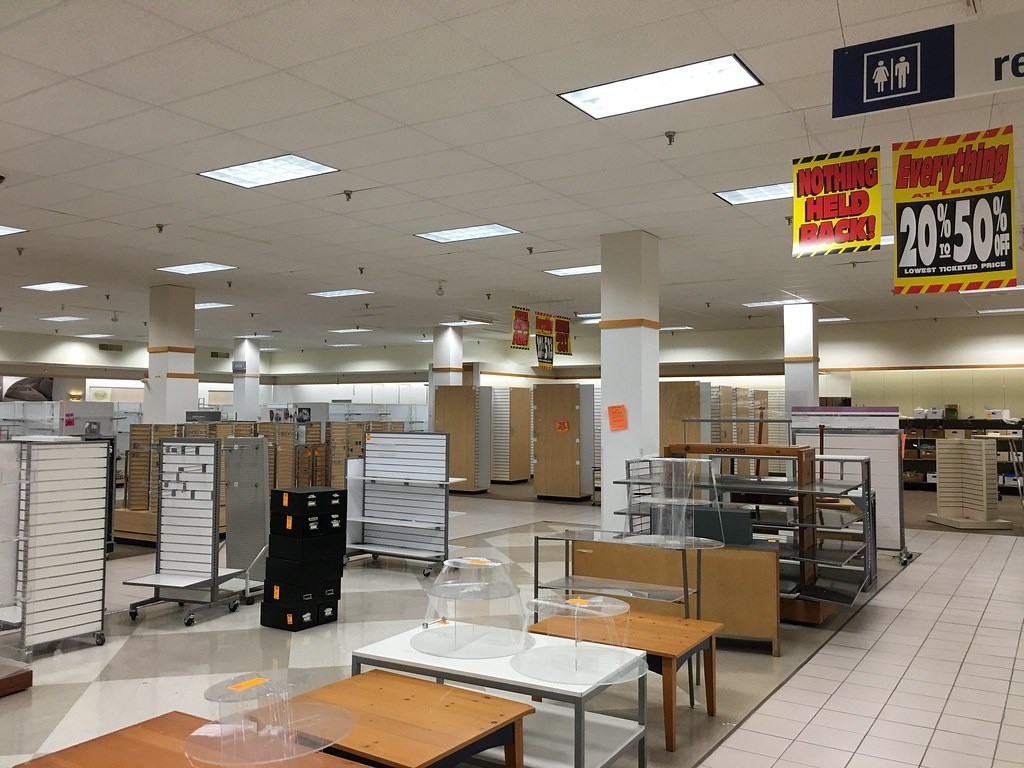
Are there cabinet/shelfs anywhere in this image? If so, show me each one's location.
[344,431,467,577]
[533,529,727,708]
[900,418,1024,496]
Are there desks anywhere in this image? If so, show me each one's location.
[351,619,648,768]
[11,710,373,768]
[527,606,724,753]
[245,668,536,768]
